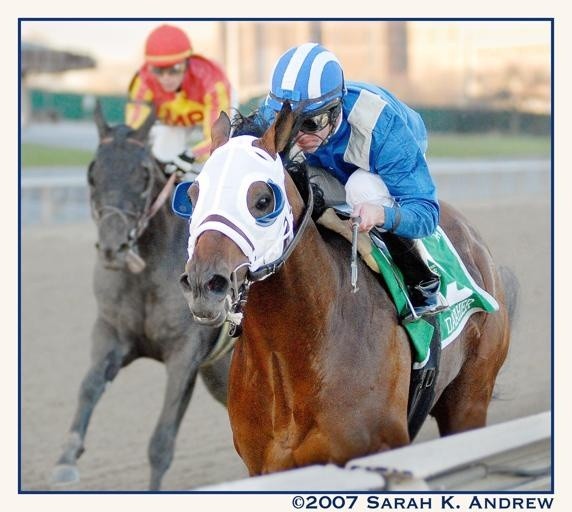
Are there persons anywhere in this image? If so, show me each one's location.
[170,42,451,325]
[123,24,239,185]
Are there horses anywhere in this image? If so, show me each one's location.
[48,97,238,490]
[181,97,518,479]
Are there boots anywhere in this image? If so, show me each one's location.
[378,230,441,320]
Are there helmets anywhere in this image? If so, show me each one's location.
[144,24,193,68]
[265,42,347,113]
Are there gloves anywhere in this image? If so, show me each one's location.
[164,151,197,177]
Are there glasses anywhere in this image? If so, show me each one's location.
[299,111,331,134]
[153,64,181,75]
